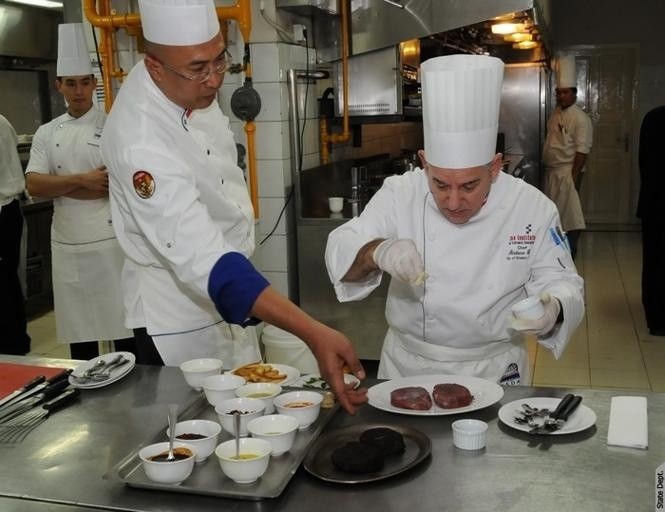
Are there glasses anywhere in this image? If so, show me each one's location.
[151,50,233,83]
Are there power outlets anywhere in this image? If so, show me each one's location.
[293,23,307,44]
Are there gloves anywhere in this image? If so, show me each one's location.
[508,293,560,335]
[373,238,425,285]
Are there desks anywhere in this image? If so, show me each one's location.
[0,354,665,512]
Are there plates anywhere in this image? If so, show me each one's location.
[498,398,598,434]
[302,423,433,485]
[227,362,300,388]
[299,371,362,392]
[68,352,136,388]
[365,374,505,416]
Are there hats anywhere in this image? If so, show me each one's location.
[420,54,505,169]
[54,21,95,77]
[138,1,221,46]
[555,53,579,90]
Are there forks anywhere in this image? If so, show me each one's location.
[76,355,129,382]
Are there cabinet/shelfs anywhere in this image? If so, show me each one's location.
[12,191,60,323]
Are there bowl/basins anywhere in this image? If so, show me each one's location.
[233,383,283,414]
[179,358,223,393]
[272,391,323,432]
[246,414,300,459]
[138,444,196,489]
[202,373,246,414]
[166,420,222,464]
[217,437,270,485]
[215,399,266,438]
[512,296,545,321]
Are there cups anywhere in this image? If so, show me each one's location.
[329,198,344,212]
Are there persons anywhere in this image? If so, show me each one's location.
[0,114,31,356]
[99,0,370,416]
[324,54,586,388]
[636,104,664,336]
[542,54,593,261]
[23,22,145,362]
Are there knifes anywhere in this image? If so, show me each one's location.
[517,394,583,429]
[1,369,70,425]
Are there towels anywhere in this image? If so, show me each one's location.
[606,395,648,450]
